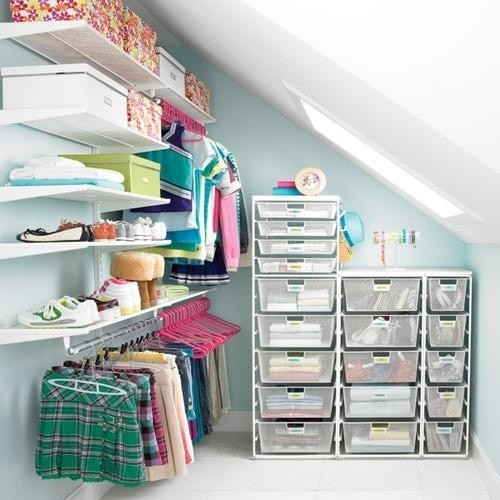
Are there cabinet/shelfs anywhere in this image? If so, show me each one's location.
[248,193,470,457]
[0,19,215,349]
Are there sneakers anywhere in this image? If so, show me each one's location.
[18,251,164,328]
[16,218,166,241]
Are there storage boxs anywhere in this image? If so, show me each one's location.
[123,83,163,146]
[342,277,420,454]
[255,202,337,454]
[425,277,467,454]
[6,0,209,109]
[53,152,162,199]
[0,63,130,131]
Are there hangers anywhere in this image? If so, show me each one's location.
[42,294,244,399]
[152,96,209,147]
